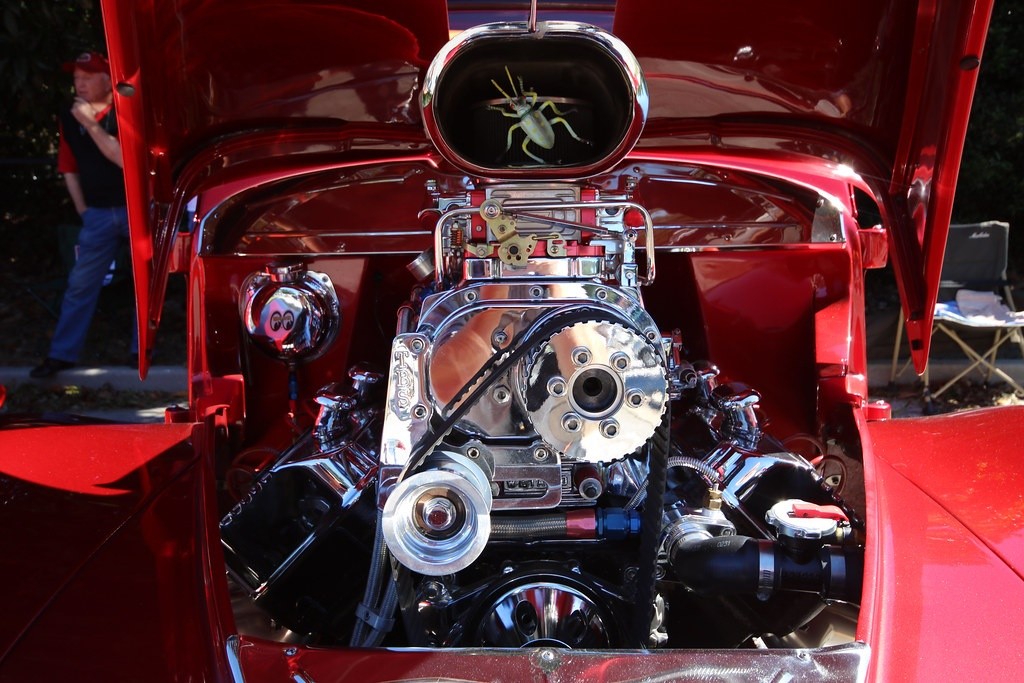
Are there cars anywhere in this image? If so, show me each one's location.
[1,1,1024,683]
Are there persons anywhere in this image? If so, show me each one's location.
[29,52,139,379]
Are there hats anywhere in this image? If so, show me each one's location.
[64,53,110,75]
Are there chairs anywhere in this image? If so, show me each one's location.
[887,220,1024,415]
